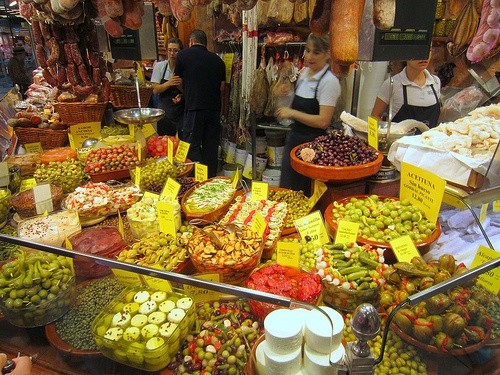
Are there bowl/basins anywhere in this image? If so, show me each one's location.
[46,278,103,358]
[324,193,442,261]
[382,301,492,355]
[90,281,197,371]
[61,224,129,278]
[324,274,378,312]
[17,208,83,248]
[187,224,263,286]
[289,135,383,183]
[230,186,313,235]
[249,333,264,375]
[180,174,237,226]
[245,261,326,317]
[0,254,77,329]
[10,183,64,218]
[224,198,289,257]
[378,119,418,137]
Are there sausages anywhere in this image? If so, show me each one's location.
[30,17,110,103]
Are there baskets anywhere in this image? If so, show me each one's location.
[14,126,69,149]
[380,299,492,356]
[51,89,109,126]
[109,62,154,110]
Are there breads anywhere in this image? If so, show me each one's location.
[421,102,500,158]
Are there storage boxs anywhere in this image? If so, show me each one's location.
[396,136,500,195]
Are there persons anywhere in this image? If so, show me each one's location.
[275,32,342,198]
[8,47,33,100]
[21,36,37,79]
[371,43,440,135]
[173,31,226,180]
[149,36,184,140]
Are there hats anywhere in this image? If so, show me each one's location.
[11,46,26,54]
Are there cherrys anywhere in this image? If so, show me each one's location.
[296,129,379,166]
